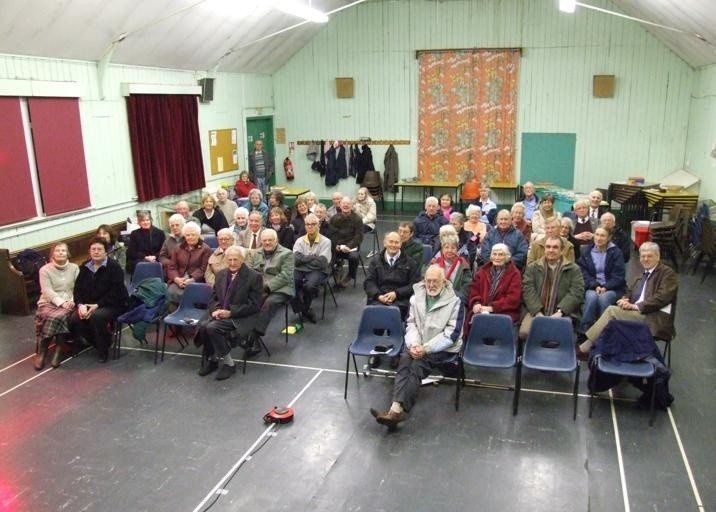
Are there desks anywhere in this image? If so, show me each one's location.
[488,183,519,203]
[266,188,310,205]
[393,180,463,214]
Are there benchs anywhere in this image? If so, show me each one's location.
[0,220,128,316]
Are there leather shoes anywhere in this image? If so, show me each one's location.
[370,407,398,429]
[215,363,237,380]
[376,409,410,425]
[341,275,354,288]
[97,348,109,363]
[245,341,263,356]
[107,338,114,347]
[301,307,312,322]
[306,308,318,324]
[575,344,590,361]
[198,360,219,376]
[368,356,382,368]
[239,334,253,349]
[390,355,400,369]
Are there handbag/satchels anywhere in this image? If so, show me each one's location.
[374,340,396,352]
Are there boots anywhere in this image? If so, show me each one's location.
[33,336,50,370]
[50,333,65,368]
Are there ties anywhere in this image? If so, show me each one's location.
[630,271,650,304]
[591,210,597,218]
[581,218,587,224]
[390,258,394,266]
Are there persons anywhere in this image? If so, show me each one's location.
[362,177,679,430]
[35,187,377,381]
[248,139,274,196]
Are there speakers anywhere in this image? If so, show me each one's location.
[199,78,214,102]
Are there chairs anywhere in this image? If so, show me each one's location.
[240,329,270,374]
[360,170,384,213]
[160,283,214,366]
[126,262,164,293]
[117,278,184,365]
[690,219,715,284]
[321,278,338,321]
[514,316,580,421]
[589,319,657,426]
[365,227,379,252]
[423,243,432,264]
[439,354,459,410]
[632,277,676,368]
[204,235,219,251]
[109,319,143,360]
[353,255,367,288]
[645,204,686,272]
[456,314,519,415]
[344,305,403,399]
[279,299,304,343]
